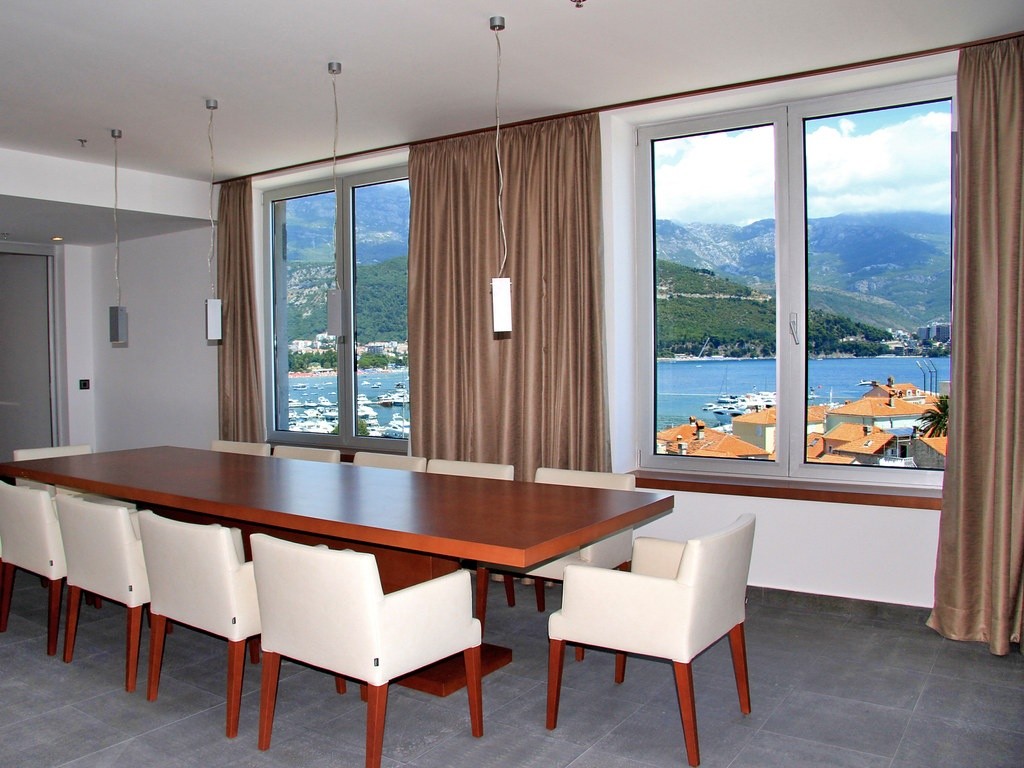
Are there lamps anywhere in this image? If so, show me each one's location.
[490,16,511,341]
[205,99,221,340]
[327,62,343,337]
[109,129,127,344]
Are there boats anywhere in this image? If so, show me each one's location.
[289,376,410,438]
[702,366,819,421]
[857,380,872,385]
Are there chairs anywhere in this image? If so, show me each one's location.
[0,440,756,768]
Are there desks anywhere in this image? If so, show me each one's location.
[0,445,674,697]
